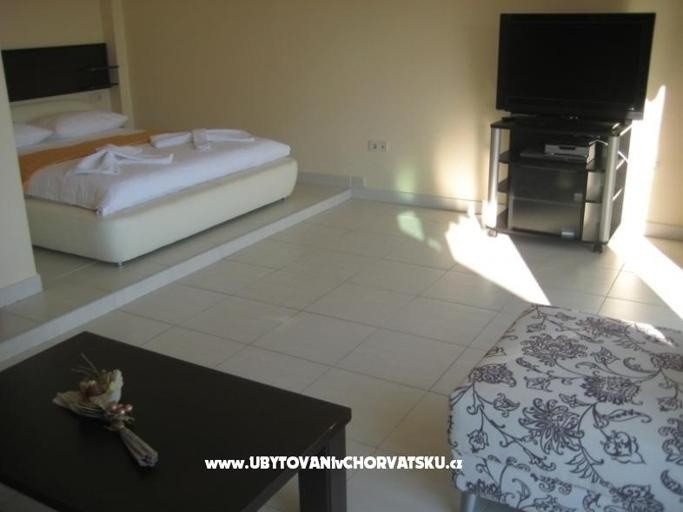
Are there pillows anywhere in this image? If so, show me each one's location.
[13,109,129,146]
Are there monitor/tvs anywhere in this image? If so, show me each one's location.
[496,11,657,130]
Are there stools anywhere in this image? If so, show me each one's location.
[443,302,683,512]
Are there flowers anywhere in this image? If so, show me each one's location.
[50,350,158,468]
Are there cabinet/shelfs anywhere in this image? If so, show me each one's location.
[481,116,631,255]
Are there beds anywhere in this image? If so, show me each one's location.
[15,129,297,269]
